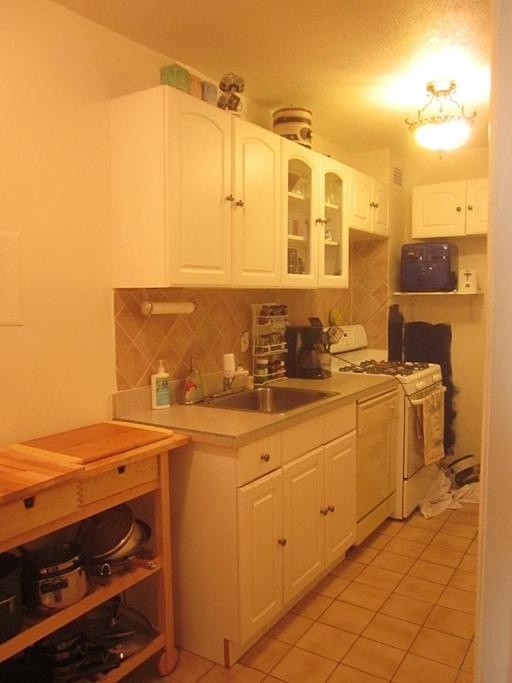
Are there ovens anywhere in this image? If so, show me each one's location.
[403,362,446,480]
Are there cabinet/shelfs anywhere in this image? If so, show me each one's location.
[356,386,400,547]
[412,178,489,239]
[350,167,389,242]
[281,399,355,619]
[1,420,190,683]
[120,433,282,668]
[107,84,280,289]
[281,136,351,290]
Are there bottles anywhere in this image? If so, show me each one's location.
[287,248,298,275]
[255,358,269,382]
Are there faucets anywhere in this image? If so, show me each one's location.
[222,371,236,393]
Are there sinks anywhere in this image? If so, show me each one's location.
[204,382,339,414]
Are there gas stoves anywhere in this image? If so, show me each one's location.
[334,355,439,379]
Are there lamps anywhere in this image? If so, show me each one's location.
[403,82,477,153]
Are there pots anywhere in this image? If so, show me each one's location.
[74,507,163,578]
[15,540,92,611]
[0,591,160,682]
